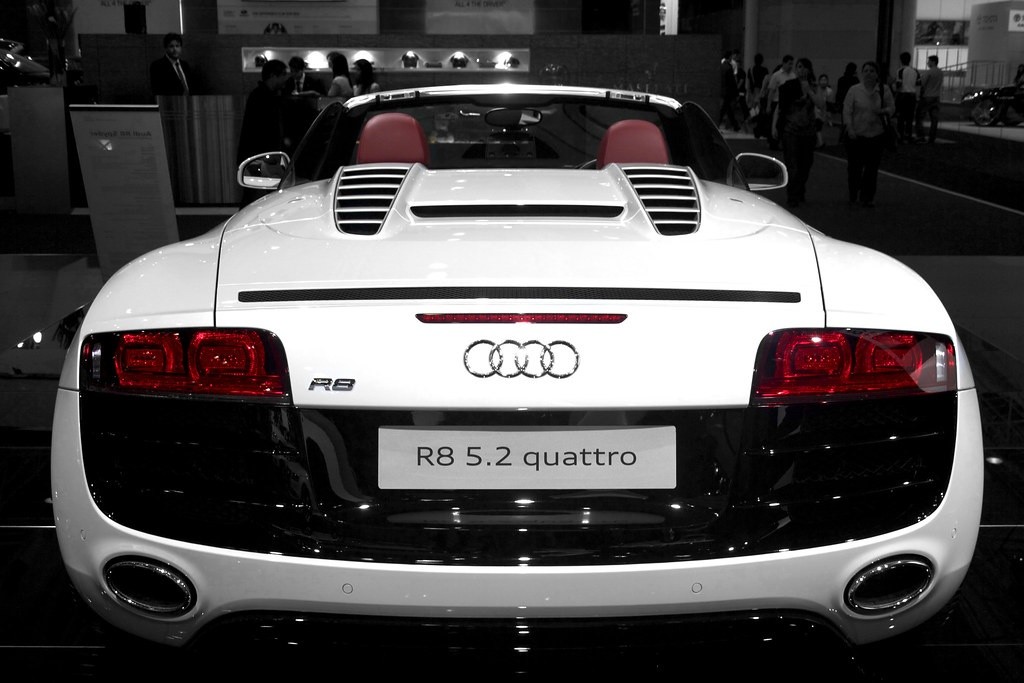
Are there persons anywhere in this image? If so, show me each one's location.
[1014,64,1024,89]
[892,52,922,146]
[915,55,943,147]
[716,49,834,152]
[149,33,212,96]
[835,61,860,157]
[842,61,896,209]
[772,57,824,209]
[237,52,383,211]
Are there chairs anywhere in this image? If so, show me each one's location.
[359,113,431,166]
[597,119,669,169]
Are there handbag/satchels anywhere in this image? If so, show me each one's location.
[883,123,898,152]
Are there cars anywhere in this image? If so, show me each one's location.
[1,50,63,145]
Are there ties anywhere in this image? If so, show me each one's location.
[296,81,300,93]
[175,62,186,89]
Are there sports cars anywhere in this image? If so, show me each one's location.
[50,81,986,661]
[958,82,1024,127]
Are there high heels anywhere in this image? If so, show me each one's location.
[815,143,826,151]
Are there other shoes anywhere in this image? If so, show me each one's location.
[900,135,914,142]
[769,145,782,152]
[916,137,934,144]
[848,201,876,212]
[787,195,806,208]
